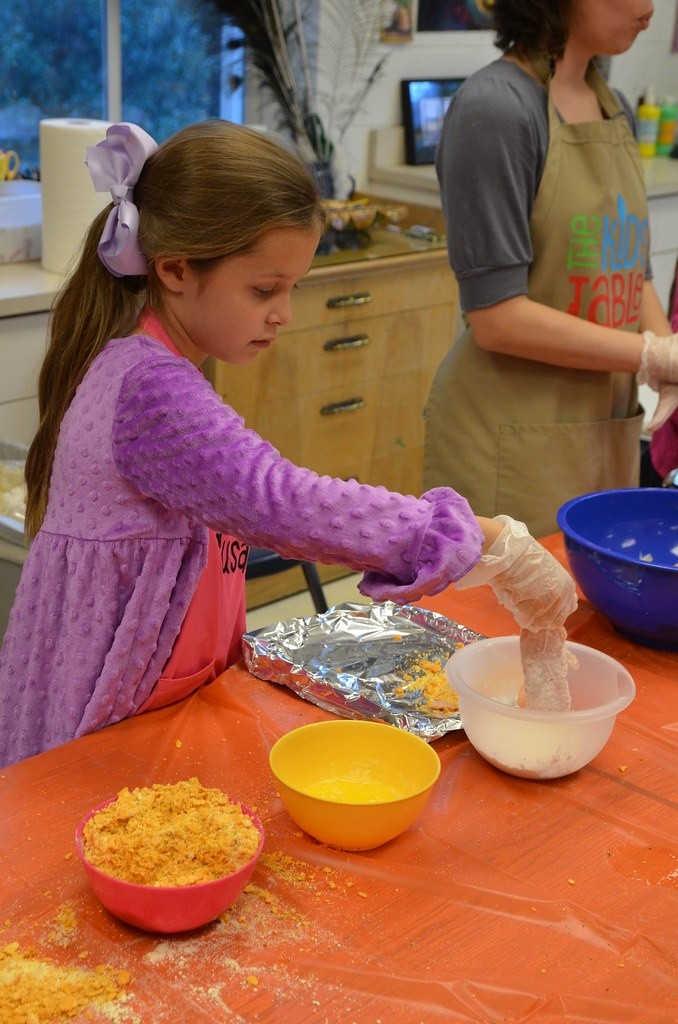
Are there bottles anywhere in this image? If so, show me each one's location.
[636,83,678,158]
[23,167,40,181]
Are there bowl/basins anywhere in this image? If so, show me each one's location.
[73,785,267,935]
[555,486,678,652]
[268,719,442,852]
[444,636,638,780]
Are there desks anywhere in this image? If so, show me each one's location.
[0,533,678,1024]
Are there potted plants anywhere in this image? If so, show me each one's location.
[211,0,404,256]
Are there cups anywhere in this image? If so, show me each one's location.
[0,149,19,185]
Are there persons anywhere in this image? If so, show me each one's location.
[0,119,578,765]
[423,0,678,543]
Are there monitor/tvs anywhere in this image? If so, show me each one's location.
[401,76,468,166]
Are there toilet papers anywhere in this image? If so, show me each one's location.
[39,118,138,274]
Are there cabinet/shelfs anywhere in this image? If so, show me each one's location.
[197,247,460,611]
